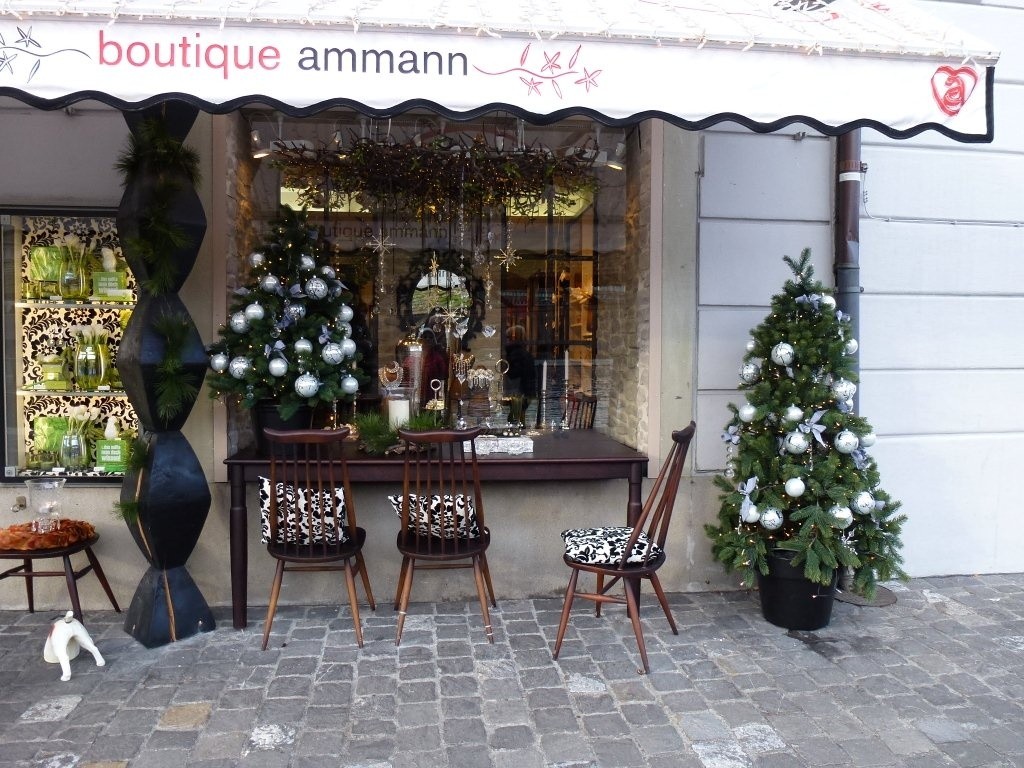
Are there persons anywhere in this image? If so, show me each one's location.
[498,325,537,396]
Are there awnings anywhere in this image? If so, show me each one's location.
[0,5,999,145]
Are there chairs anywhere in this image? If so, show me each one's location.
[548,419,696,676]
[390,429,497,646]
[259,428,375,654]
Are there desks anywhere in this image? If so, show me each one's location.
[226,427,649,634]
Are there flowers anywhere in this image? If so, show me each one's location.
[55,233,89,262]
[27,307,132,472]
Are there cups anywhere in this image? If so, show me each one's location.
[24,477,66,534]
[57,262,90,302]
[60,434,89,469]
[74,343,110,389]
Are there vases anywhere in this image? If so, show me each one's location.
[58,262,89,300]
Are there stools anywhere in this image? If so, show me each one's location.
[1,532,122,626]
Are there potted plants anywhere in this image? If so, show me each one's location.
[201,201,374,458]
[700,243,911,641]
